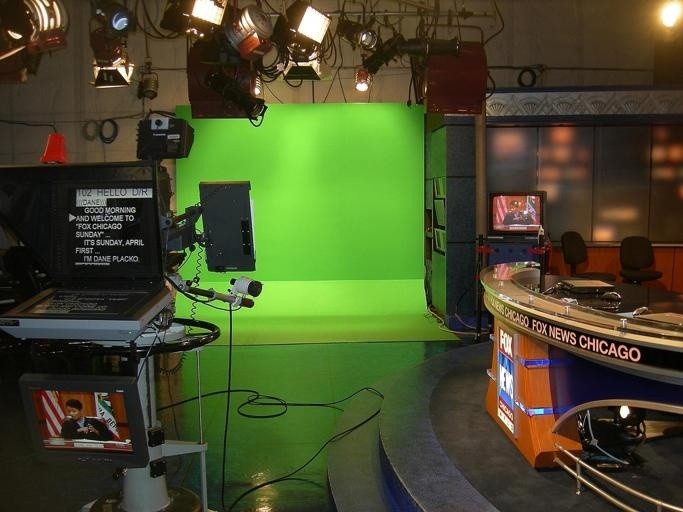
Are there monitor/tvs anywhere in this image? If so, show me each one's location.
[485,191,548,236]
[20,372,150,469]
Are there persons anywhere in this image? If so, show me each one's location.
[60,399,112,441]
[503,200,531,225]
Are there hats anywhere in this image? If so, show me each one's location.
[510,200,519,207]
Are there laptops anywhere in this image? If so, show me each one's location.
[561,279,614,294]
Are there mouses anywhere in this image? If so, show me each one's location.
[632,305,649,318]
[600,289,621,304]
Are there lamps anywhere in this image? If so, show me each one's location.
[355,69,371,93]
[283,57,321,79]
[92,59,135,87]
[292,5,333,44]
[190,0,227,29]
[336,20,377,49]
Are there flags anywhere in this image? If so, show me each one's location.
[496,196,507,225]
[525,196,537,225]
[94,392,120,442]
[36,390,66,439]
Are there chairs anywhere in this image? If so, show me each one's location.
[620,235,662,285]
[561,230,618,282]
